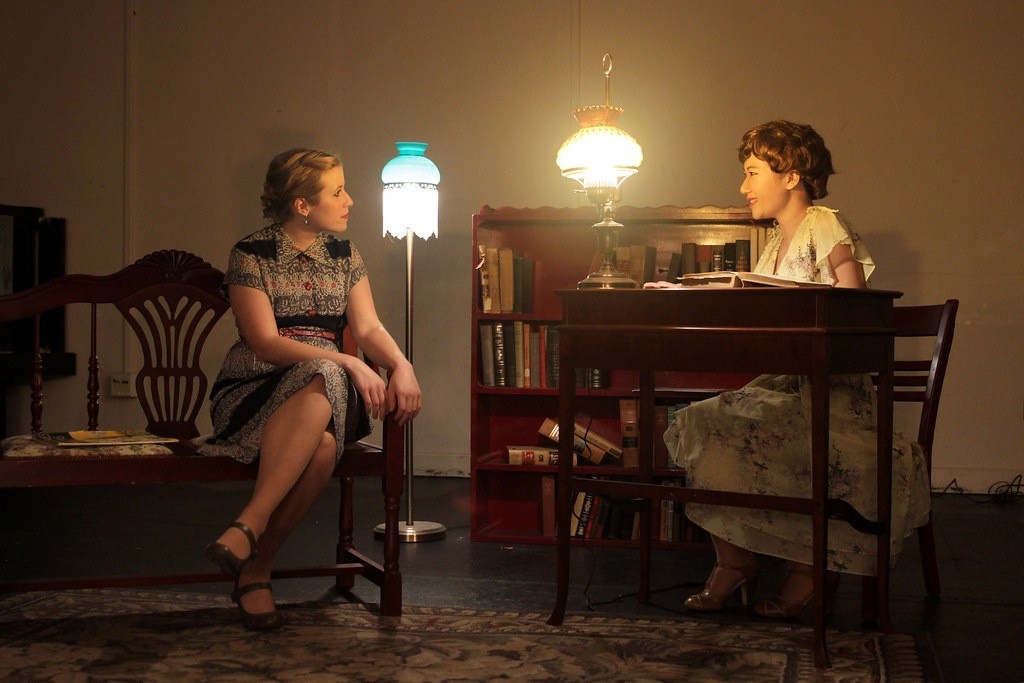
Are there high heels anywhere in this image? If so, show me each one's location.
[754,568,817,618]
[684,558,759,610]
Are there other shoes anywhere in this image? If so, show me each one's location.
[205,522,257,578]
[231,574,285,631]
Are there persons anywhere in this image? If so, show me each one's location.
[197,148,423,633]
[660,119,934,624]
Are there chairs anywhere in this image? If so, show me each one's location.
[747,298,959,634]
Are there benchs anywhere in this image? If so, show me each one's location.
[1,249,405,616]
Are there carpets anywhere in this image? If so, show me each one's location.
[0,589,943,682]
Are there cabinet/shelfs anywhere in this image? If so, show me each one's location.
[471,204,778,552]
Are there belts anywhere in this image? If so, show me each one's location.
[278,329,338,342]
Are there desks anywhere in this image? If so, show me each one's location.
[546,289,904,669]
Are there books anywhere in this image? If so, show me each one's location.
[477,246,534,313]
[508,400,707,470]
[27,428,180,446]
[566,477,702,540]
[677,268,833,291]
[478,320,612,390]
[590,239,750,290]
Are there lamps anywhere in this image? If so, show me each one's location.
[373,142,447,544]
[556,53,643,288]
